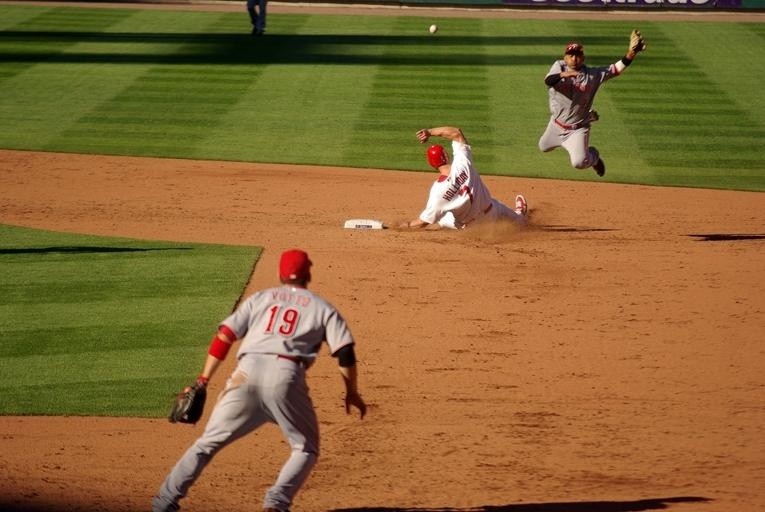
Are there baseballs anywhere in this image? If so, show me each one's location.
[429,24,438,32]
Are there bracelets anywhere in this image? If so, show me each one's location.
[621,55,632,66]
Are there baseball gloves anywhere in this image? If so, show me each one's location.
[629,29,646,56]
[168,383,206,424]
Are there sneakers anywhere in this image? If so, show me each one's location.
[515,195,528,220]
[588,146,605,177]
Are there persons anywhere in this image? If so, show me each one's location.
[247,0,267,35]
[150,250,366,512]
[387,127,527,231]
[539,29,646,177]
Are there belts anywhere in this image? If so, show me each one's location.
[553,119,585,131]
[462,202,496,226]
[278,353,305,369]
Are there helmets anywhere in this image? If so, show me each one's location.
[426,144,450,169]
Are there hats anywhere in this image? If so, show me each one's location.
[564,42,584,59]
[279,249,314,283]
